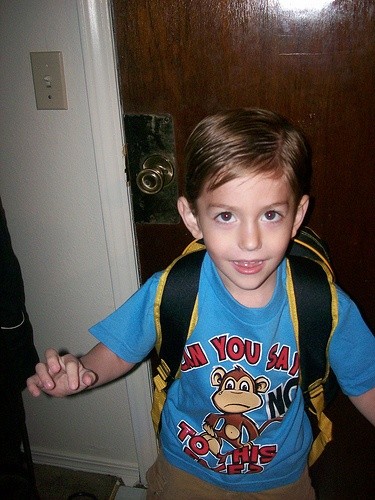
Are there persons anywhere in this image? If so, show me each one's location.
[26,109,375,500]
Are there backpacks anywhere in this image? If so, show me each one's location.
[149,226,337,469]
